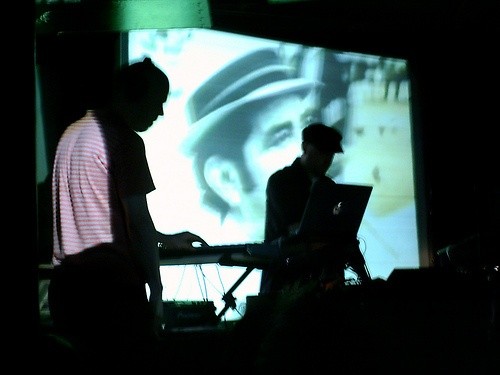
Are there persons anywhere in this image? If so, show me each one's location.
[47,56,209,353]
[182,48,327,226]
[259,123,365,330]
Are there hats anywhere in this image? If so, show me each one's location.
[181,47,313,157]
[302,123,344,153]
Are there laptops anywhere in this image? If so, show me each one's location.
[266,182,373,245]
